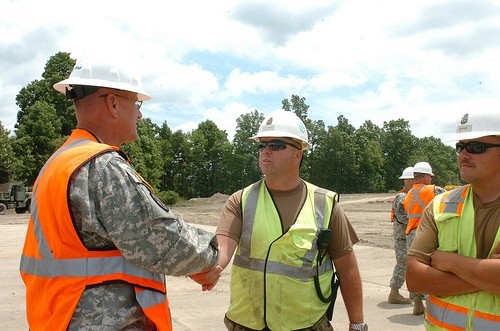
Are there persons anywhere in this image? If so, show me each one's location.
[189,110,369,331]
[389,104,500,330]
[19,45,222,331]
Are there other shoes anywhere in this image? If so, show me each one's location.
[412,298,425,316]
[388,289,411,304]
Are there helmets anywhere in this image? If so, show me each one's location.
[410,162,434,176]
[399,166,414,179]
[249,110,311,150]
[53,58,152,103]
[443,111,500,142]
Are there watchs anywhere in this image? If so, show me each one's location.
[348,323,368,331]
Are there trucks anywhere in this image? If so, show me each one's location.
[0,183,32,215]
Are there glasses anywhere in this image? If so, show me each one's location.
[99,93,143,111]
[455,141,500,154]
[259,140,300,151]
